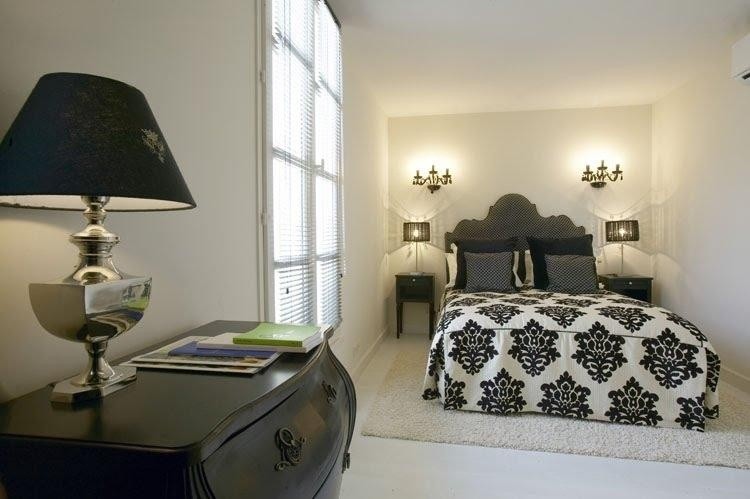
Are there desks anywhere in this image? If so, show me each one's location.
[1,321,356,498]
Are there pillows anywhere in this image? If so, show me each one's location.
[525,234,593,290]
[524,250,535,287]
[543,253,599,293]
[442,251,523,290]
[449,236,519,290]
[464,252,517,293]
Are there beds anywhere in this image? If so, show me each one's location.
[421,194,721,432]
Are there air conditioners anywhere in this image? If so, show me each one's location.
[729,33,750,85]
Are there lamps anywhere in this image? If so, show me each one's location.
[402,221,431,274]
[582,160,623,189]
[0,72,196,405]
[414,165,452,194]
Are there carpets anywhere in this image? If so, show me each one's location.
[362,335,750,471]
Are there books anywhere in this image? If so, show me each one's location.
[118,322,323,375]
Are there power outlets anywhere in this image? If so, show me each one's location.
[606,219,640,276]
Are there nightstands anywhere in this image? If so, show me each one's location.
[599,274,653,303]
[395,271,433,342]
